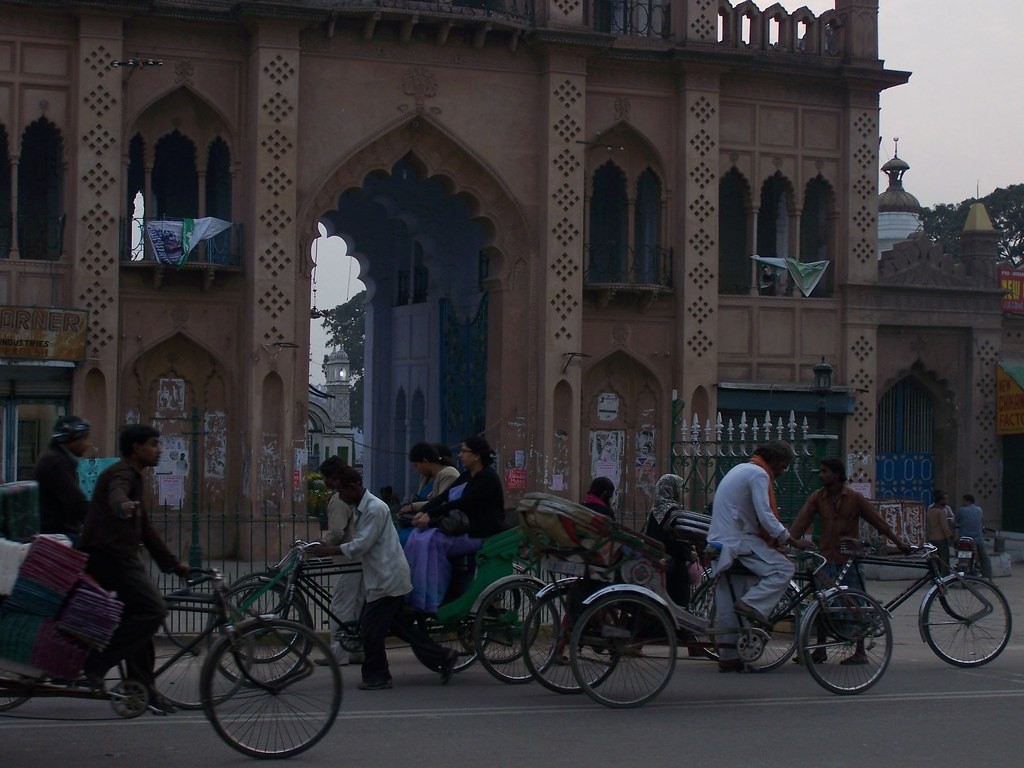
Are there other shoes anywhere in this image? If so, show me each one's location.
[315,658,350,666]
[85,663,104,690]
[793,652,828,664]
[840,652,869,666]
[988,582,999,587]
[687,642,715,657]
[148,688,177,713]
[633,646,647,658]
[544,651,572,667]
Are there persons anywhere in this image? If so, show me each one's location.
[707,441,815,674]
[315,453,459,692]
[925,490,994,585]
[546,476,622,665]
[89,423,184,716]
[399,436,509,610]
[34,413,90,536]
[788,459,912,669]
[628,474,716,657]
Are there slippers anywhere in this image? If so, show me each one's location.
[733,607,772,627]
[719,660,752,672]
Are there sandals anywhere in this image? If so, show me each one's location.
[440,649,458,685]
[357,677,392,690]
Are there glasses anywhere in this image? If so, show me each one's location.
[460,448,473,454]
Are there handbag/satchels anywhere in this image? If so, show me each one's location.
[396,494,425,528]
[437,509,469,536]
[987,552,1011,577]
[687,558,705,586]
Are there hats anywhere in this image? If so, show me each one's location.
[51,415,91,443]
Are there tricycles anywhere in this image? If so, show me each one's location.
[206,491,1012,708]
[0,566,343,760]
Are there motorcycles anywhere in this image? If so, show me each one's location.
[954,525,997,588]
